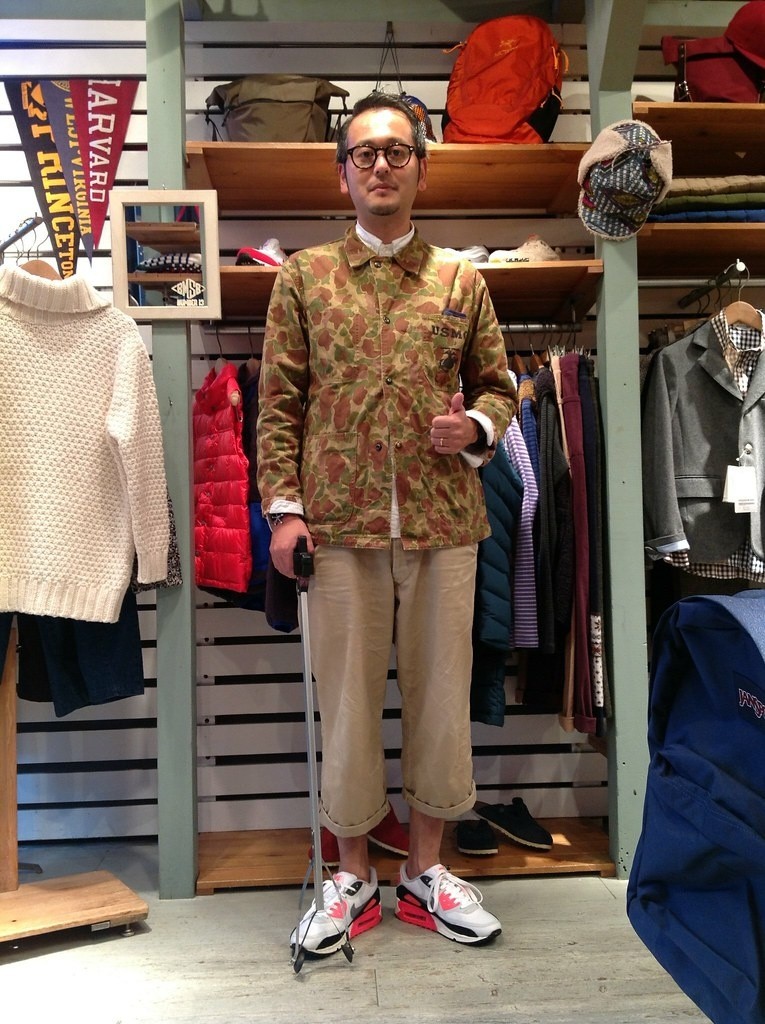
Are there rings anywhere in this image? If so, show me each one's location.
[438,438,443,447]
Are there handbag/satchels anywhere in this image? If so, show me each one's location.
[661,36,765,103]
[206,74,349,142]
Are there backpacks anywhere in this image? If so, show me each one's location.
[441,14,569,144]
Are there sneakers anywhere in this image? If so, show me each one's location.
[289,867,382,955]
[487,238,562,262]
[235,238,288,267]
[394,863,503,945]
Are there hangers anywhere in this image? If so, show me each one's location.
[1,216,63,282]
[639,263,764,352]
[215,325,590,387]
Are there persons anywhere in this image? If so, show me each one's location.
[254,92,519,961]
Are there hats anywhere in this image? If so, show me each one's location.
[577,120,673,239]
[723,0,765,68]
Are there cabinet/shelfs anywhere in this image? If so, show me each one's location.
[632,103,765,256]
[126,222,202,290]
[185,141,604,323]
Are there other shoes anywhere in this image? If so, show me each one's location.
[308,827,341,866]
[470,797,554,849]
[366,802,410,855]
[444,246,488,263]
[455,821,499,854]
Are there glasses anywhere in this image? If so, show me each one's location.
[347,144,418,170]
